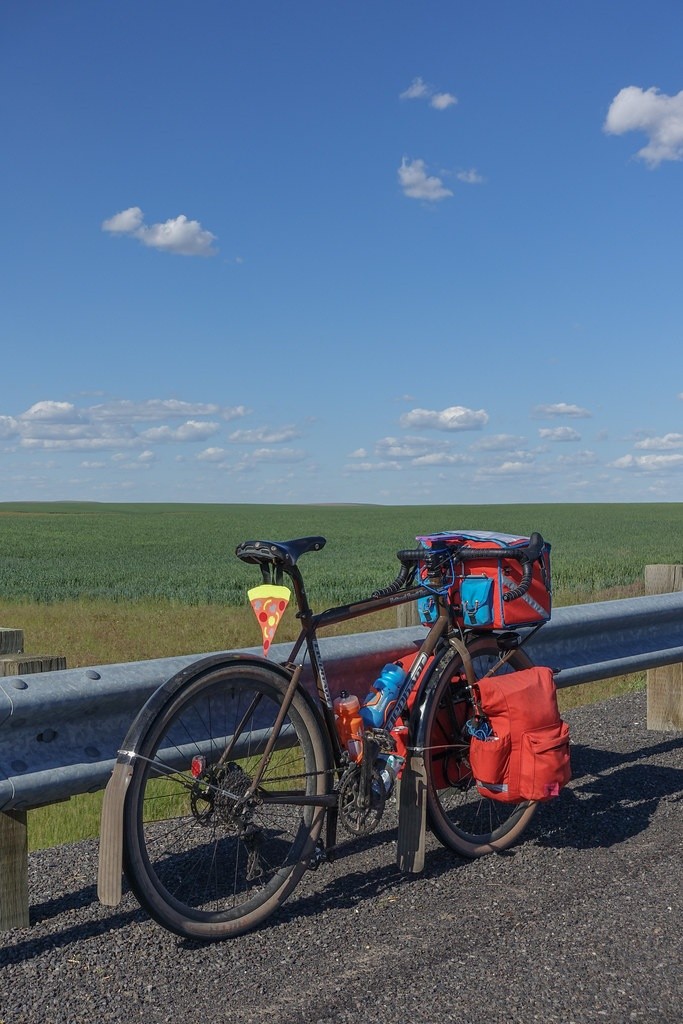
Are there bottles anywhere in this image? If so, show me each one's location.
[358,660,408,728]
[332,688,362,764]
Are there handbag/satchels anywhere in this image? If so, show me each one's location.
[413,527,553,630]
[468,663,572,803]
[392,646,476,793]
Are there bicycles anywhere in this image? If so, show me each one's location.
[95,518,573,943]
